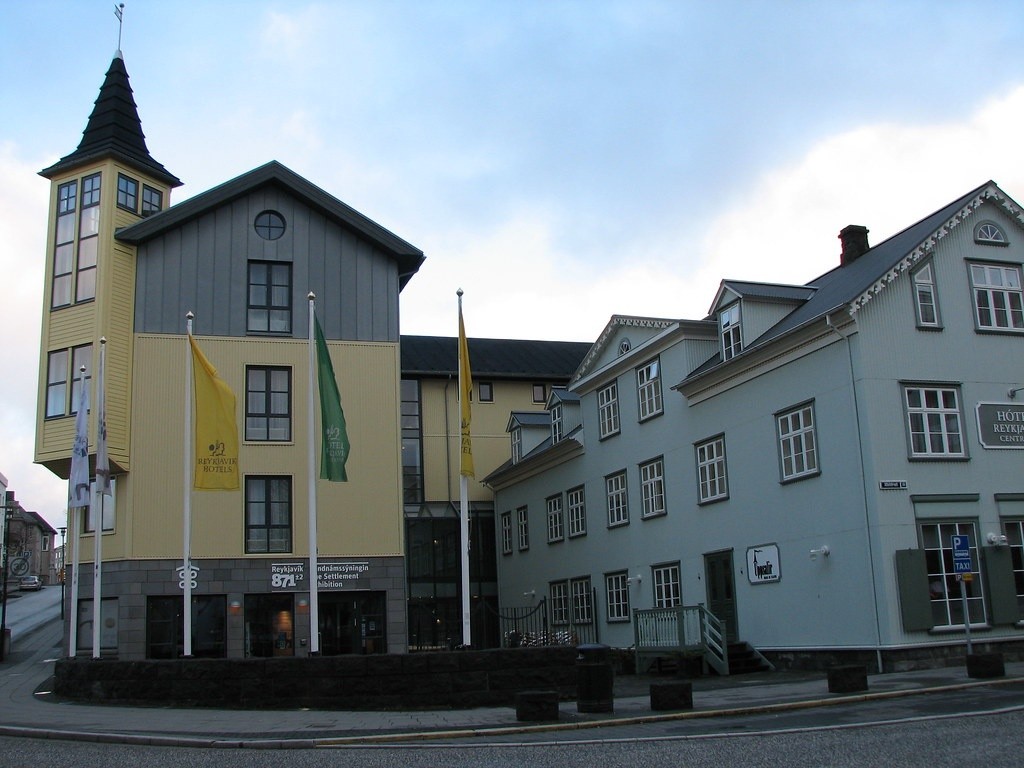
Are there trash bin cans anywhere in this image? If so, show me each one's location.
[573,641,615,714]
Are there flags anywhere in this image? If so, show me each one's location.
[314,312,349,482]
[458,310,475,479]
[68,373,92,508]
[188,333,240,491]
[96,350,112,497]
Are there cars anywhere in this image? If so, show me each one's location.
[20,576,43,591]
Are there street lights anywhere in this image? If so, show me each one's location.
[57,527,67,620]
[0,505,22,660]
